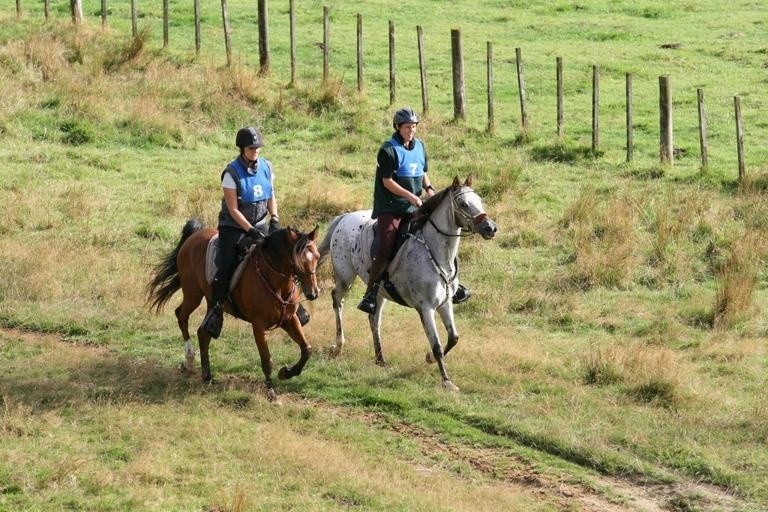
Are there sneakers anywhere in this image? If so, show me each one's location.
[207,312,223,338]
[297,304,311,325]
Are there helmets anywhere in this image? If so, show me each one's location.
[236,128,264,148]
[393,109,419,128]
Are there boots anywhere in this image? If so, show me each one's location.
[453,288,469,304]
[361,256,389,311]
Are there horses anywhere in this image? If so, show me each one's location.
[294,171,499,395]
[145,217,321,407]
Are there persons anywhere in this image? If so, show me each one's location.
[205,126,309,339]
[359,108,471,314]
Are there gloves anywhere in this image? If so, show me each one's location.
[269,219,279,234]
[247,227,266,240]
